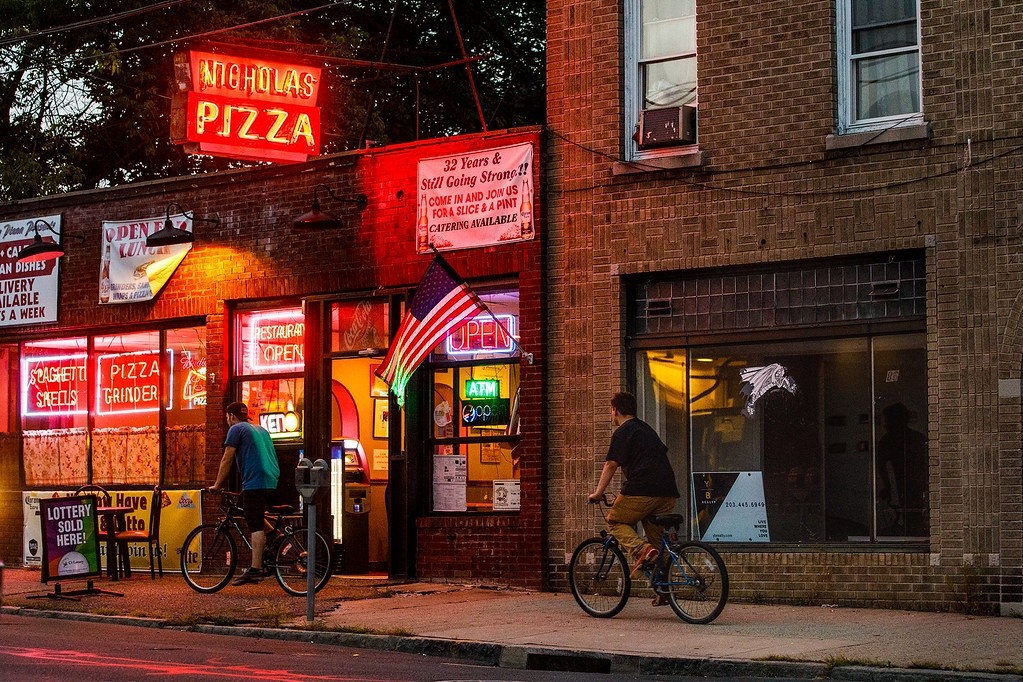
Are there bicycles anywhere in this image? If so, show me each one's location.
[181,487,333,596]
[569,492,730,624]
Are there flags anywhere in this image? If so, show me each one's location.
[374,253,489,398]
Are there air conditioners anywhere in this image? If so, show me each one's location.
[639,105,696,147]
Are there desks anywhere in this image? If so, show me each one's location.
[97,506,135,581]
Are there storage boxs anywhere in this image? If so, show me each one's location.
[492,479,521,511]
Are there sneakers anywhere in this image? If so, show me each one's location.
[630,545,659,577]
[231,567,265,587]
[650,595,670,607]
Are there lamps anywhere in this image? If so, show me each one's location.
[291,184,368,230]
[17,219,86,263]
[146,203,219,248]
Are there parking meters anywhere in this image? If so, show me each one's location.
[295,459,329,622]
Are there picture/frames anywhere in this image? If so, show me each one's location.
[480,430,501,463]
[370,363,390,398]
[373,399,389,439]
[471,353,511,435]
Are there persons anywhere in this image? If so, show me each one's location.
[208,402,280,586]
[589,392,679,607]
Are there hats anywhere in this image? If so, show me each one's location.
[227,403,254,422]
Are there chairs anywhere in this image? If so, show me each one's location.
[72,484,163,581]
